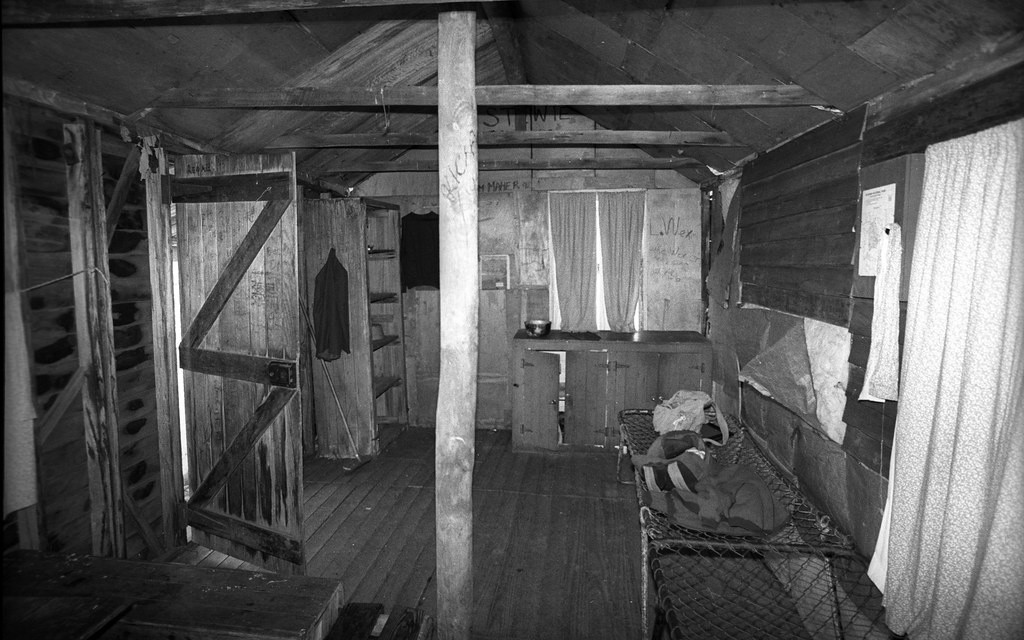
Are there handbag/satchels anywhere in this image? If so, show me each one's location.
[652,389,729,446]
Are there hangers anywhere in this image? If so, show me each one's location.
[412,201,431,214]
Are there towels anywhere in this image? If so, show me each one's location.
[855,221,905,403]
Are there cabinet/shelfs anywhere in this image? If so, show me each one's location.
[510,328,714,458]
[301,196,411,460]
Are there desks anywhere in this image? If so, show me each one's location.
[0,546,345,640]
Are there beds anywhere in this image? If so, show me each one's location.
[615,400,910,640]
